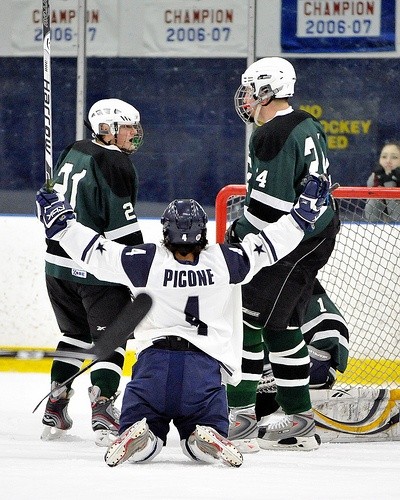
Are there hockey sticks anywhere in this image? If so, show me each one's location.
[0,293,154,362]
[41,0,54,188]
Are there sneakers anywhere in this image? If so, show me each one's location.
[88,385,321,468]
[40,382,83,442]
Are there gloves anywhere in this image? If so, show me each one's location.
[374,164,386,182]
[389,166,400,184]
[35,188,75,238]
[290,172,330,232]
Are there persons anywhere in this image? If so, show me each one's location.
[222,57,342,451]
[35,174,340,470]
[254,279,350,419]
[39,98,144,446]
[364,143,400,223]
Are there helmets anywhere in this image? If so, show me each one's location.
[161,199,209,244]
[88,98,140,138]
[241,57,296,98]
